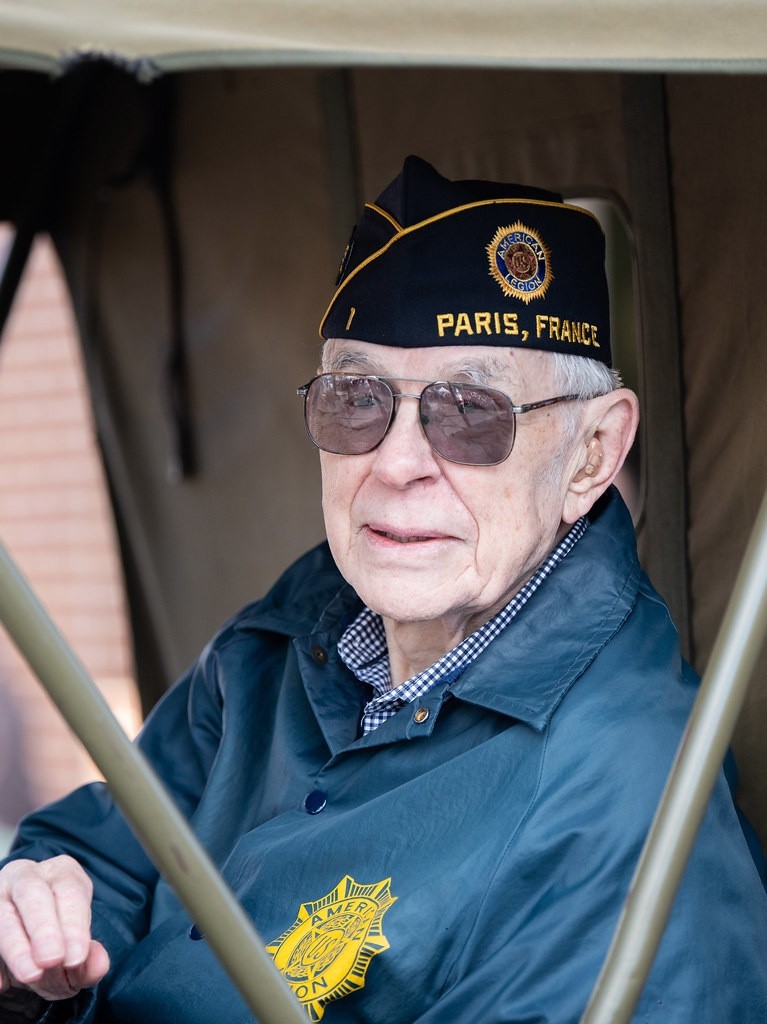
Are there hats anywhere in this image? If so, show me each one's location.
[317,155,612,367]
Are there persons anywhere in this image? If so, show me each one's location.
[0,152,767,1024]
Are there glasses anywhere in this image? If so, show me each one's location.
[297,371,607,467]
